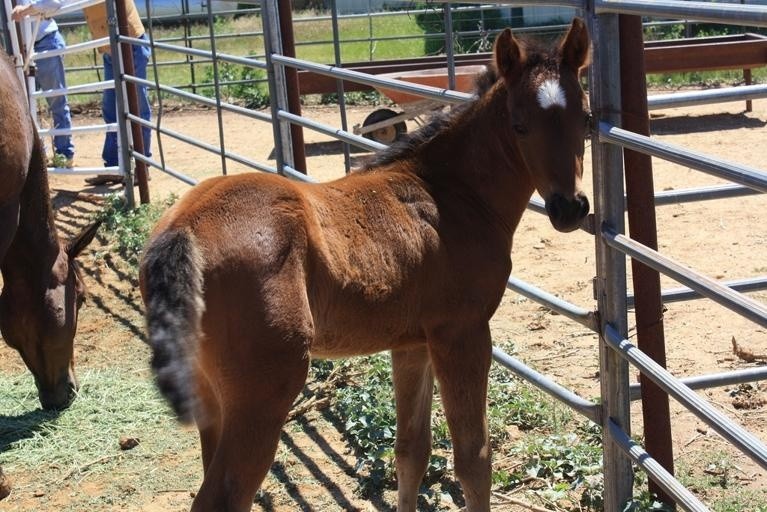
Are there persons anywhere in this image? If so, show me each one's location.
[14,0,75,168]
[10,0,156,186]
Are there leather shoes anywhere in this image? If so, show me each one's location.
[85,174,125,186]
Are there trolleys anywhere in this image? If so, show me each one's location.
[353,63,488,150]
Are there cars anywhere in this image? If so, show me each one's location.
[414,0,582,52]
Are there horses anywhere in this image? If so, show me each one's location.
[136,15,596,512]
[0,46,104,500]
